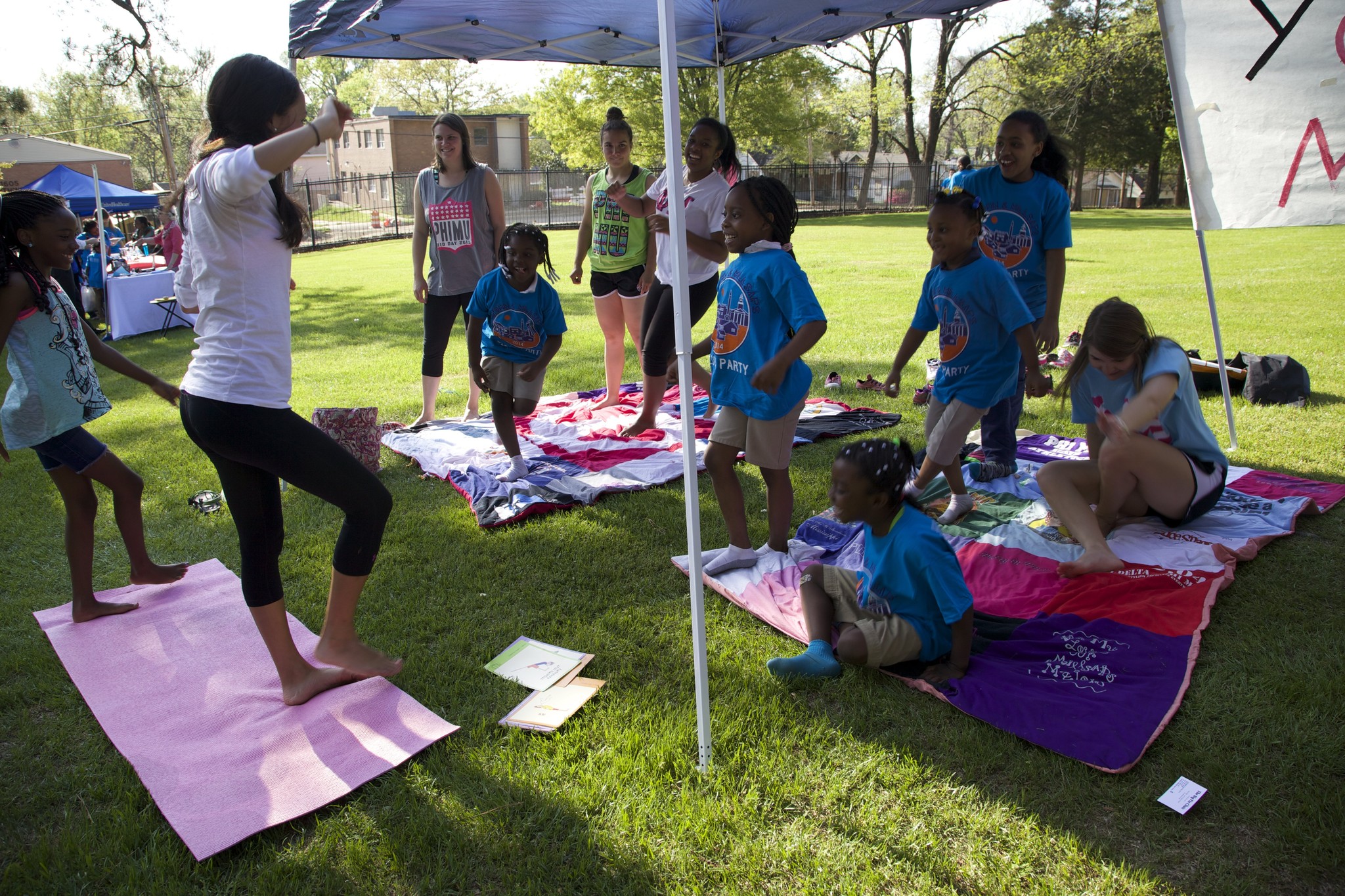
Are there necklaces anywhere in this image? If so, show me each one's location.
[685,173,692,184]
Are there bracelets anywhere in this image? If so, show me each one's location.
[306,122,320,147]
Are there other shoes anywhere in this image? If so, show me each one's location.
[85,312,106,335]
[823,329,1081,404]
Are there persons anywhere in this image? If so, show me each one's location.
[411,114,507,434]
[169,52,409,710]
[948,154,979,194]
[51,205,185,336]
[465,221,570,483]
[941,168,956,192]
[956,109,1073,481]
[1035,296,1228,580]
[568,104,662,411]
[764,437,975,687]
[606,116,744,439]
[665,175,829,576]
[882,188,1054,528]
[1,189,187,623]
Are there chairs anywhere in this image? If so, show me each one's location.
[150,291,195,338]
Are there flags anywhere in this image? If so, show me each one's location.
[1156,0,1345,235]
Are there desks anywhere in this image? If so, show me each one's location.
[107,269,199,342]
[105,254,168,273]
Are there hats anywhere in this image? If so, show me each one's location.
[154,205,175,216]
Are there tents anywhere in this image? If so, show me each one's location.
[0,130,162,338]
[289,0,1011,779]
